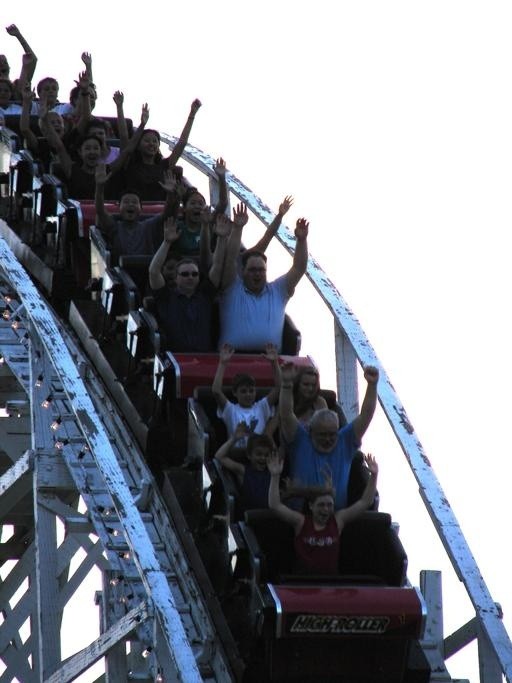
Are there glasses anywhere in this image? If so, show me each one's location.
[178,270,200,278]
[248,266,266,273]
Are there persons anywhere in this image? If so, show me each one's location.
[0,24,378,585]
